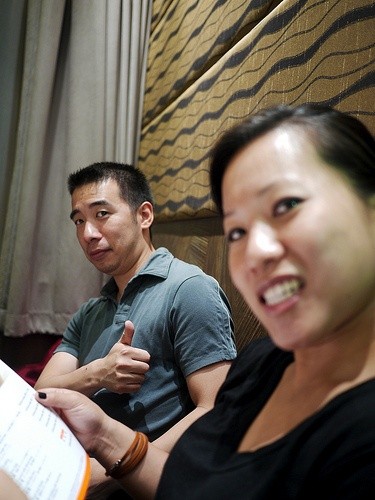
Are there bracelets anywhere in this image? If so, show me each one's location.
[105,431,148,482]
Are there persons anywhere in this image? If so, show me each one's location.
[0,101,375,500]
[33,160,236,500]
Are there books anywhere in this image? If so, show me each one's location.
[0,360,92,500]
